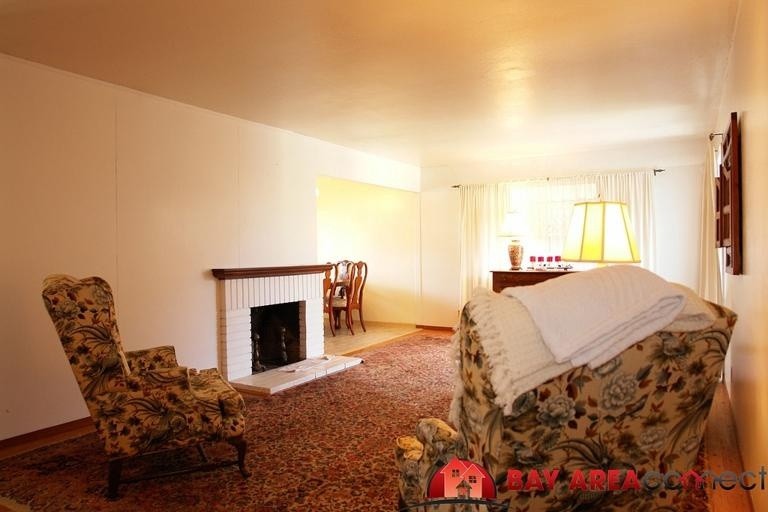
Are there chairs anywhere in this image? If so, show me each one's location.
[42,273,251,501]
[394,299,739,512]
[323,260,368,336]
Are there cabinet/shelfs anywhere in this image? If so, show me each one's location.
[490,273,577,293]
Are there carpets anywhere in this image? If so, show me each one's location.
[0,330,705,512]
[324,318,423,357]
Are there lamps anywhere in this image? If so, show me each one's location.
[500,211,530,270]
[560,194,642,267]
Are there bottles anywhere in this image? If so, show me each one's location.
[508,240,524,270]
[529,255,562,269]
